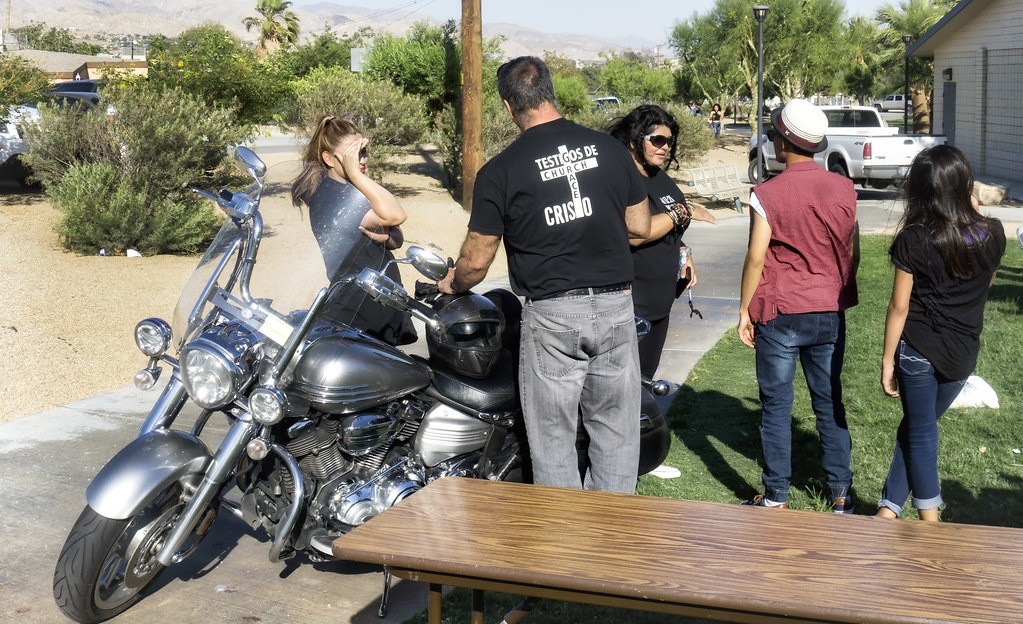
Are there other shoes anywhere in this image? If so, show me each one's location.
[831,493,854,513]
[741,493,789,508]
[649,464,682,479]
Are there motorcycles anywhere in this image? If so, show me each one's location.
[51,145,673,624]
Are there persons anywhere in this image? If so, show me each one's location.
[710,104,722,142]
[437,56,650,495]
[873,145,1007,522]
[290,115,419,349]
[607,104,716,479]
[735,99,859,515]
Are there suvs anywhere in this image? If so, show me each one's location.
[0,78,130,195]
[590,96,620,122]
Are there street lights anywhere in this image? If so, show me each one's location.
[751,5,770,187]
[902,34,912,134]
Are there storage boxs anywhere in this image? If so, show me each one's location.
[520,379,671,477]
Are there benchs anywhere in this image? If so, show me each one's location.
[687,163,750,213]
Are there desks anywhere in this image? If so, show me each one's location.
[331,477,1023,624]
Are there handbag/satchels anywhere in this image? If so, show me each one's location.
[947,374,1001,410]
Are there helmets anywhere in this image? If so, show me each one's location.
[425,289,505,379]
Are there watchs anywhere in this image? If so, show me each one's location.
[449,279,455,292]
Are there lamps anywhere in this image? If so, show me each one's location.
[942,67,952,82]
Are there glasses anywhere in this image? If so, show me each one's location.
[766,129,777,142]
[643,134,676,148]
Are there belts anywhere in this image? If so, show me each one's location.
[550,283,630,298]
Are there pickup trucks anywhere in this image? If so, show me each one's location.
[874,94,913,112]
[746,103,948,187]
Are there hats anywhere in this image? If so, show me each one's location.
[770,99,829,153]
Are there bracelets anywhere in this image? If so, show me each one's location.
[661,201,694,227]
